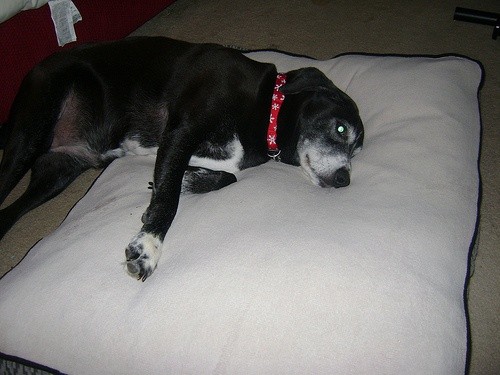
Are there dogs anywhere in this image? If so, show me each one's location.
[0,37,364,282]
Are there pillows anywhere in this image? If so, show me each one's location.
[2,49,485,374]
